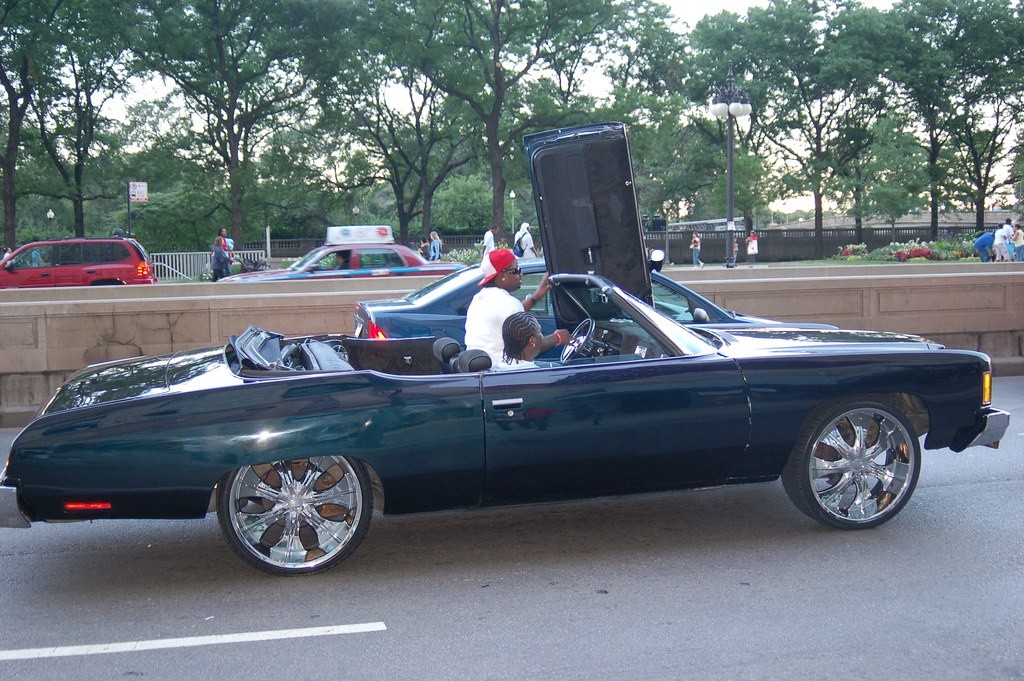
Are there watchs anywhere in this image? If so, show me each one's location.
[526,294,536,303]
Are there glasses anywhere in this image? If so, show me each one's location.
[503,266,521,274]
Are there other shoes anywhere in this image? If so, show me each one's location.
[700,263,703,268]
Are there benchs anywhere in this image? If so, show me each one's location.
[301,340,354,371]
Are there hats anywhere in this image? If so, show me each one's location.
[478,247,515,286]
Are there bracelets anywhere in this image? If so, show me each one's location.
[556,334,562,346]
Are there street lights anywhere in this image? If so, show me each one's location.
[709,59,752,269]
[352,204,360,224]
[47,209,55,229]
[509,190,516,234]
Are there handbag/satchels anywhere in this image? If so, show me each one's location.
[514,232,528,257]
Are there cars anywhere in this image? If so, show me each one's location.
[218,224,470,280]
[353,257,841,359]
[0,234,160,290]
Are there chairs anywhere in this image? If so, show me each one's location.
[383,251,403,267]
[432,337,492,374]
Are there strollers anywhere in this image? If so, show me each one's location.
[234,254,272,273]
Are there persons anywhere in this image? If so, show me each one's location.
[1012,224,1024,261]
[31,249,43,267]
[465,249,569,365]
[1002,218,1014,262]
[689,232,704,269]
[746,230,758,269]
[734,237,739,266]
[974,232,995,262]
[491,312,544,368]
[993,224,1008,262]
[419,236,430,261]
[430,231,442,261]
[210,236,233,282]
[515,223,539,259]
[218,228,233,276]
[3,247,12,267]
[328,253,348,269]
[480,225,499,263]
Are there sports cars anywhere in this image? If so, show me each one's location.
[0,120,1014,578]
